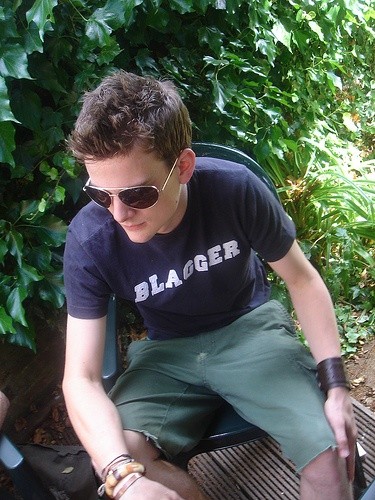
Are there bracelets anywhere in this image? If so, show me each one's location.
[98,454,146,500]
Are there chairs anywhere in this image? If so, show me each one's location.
[100,291,273,473]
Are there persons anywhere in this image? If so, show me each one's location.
[60,69,359,500]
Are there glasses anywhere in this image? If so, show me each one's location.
[82,150,180,211]
